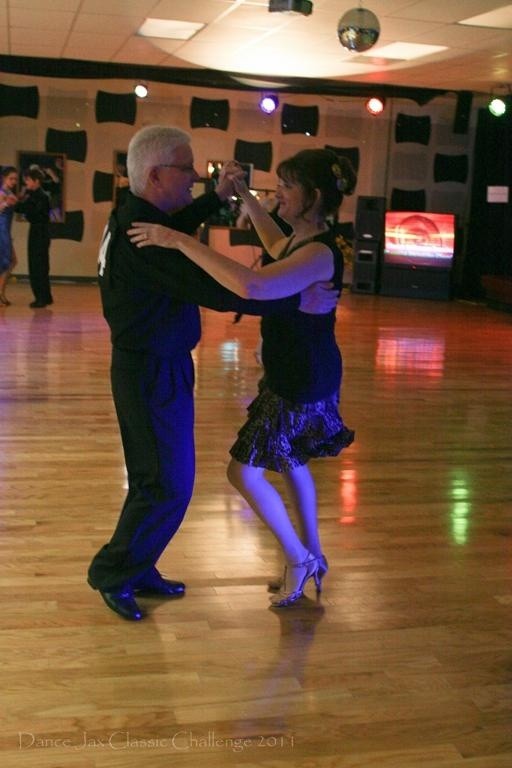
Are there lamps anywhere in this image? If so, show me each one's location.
[258,91,279,115]
[337,0,382,53]
[363,92,387,118]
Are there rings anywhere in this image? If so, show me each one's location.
[142,230,150,241]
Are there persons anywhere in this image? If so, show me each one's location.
[1,162,23,309]
[2,167,59,308]
[119,143,363,611]
[83,120,343,628]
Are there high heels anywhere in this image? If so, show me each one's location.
[270,550,320,607]
[268,554,327,592]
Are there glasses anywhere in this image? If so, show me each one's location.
[159,162,195,176]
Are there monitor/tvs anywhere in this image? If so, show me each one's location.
[483,183,511,205]
[383,210,458,272]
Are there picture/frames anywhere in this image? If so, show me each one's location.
[13,148,68,226]
[205,158,254,233]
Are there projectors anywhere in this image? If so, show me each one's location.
[268,0,313,17]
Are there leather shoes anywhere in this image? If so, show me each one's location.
[135,566,184,596]
[87,575,143,620]
[31,299,45,308]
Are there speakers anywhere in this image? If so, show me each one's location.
[355,196,386,242]
[353,241,379,294]
